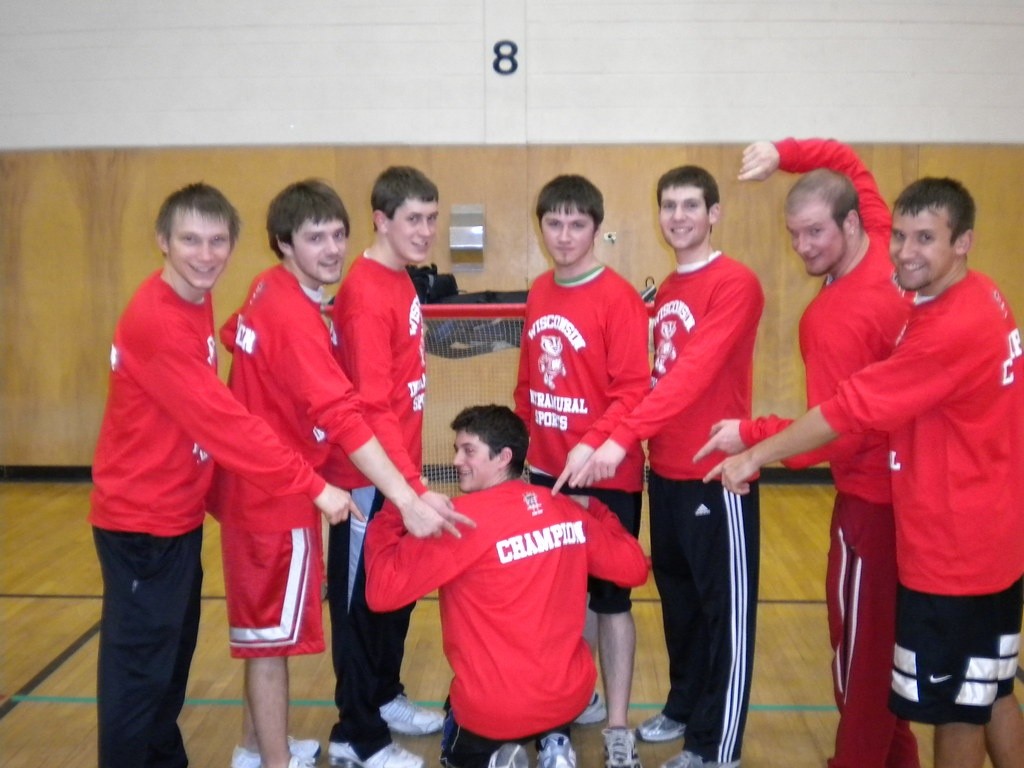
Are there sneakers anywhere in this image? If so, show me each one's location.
[601,727,642,768]
[328,731,425,768]
[379,694,447,735]
[660,750,741,768]
[537,732,577,768]
[231,736,321,768]
[488,742,529,768]
[636,714,686,742]
[572,693,608,725]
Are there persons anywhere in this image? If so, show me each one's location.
[86,182,365,768]
[323,166,479,768]
[204,178,461,768]
[702,178,1024,768]
[514,173,648,768]
[689,139,920,768]
[570,164,763,768]
[364,405,654,768]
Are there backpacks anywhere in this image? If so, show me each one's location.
[406,263,461,346]
